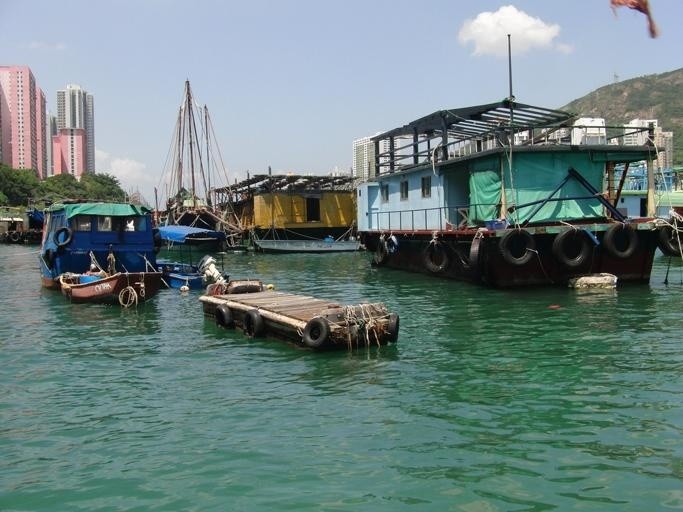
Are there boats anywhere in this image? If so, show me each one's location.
[200,275,401,351]
[0,202,44,246]
[604,164,683,224]
[151,225,231,292]
[252,232,362,253]
[56,268,166,305]
[652,191,683,258]
[40,198,157,292]
[354,33,683,291]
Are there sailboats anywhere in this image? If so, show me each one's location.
[152,80,242,252]
